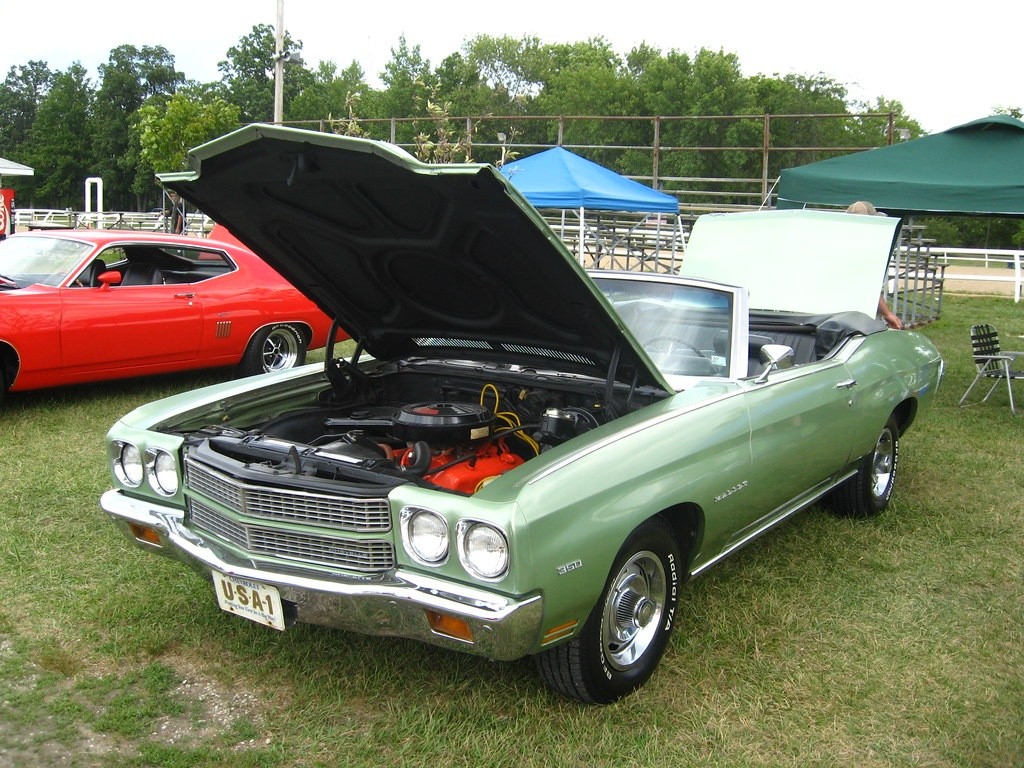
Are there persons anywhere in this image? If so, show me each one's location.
[158,192,187,234]
[845,200,910,329]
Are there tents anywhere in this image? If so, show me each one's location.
[776,116,1024,252]
[498,145,679,276]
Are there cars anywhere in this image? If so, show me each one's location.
[0,222,355,402]
[98,122,947,710]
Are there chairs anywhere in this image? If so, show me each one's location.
[713,330,774,375]
[74,259,106,287]
[958,324,1024,416]
[119,260,163,286]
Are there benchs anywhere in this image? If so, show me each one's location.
[163,269,223,283]
[661,321,817,365]
